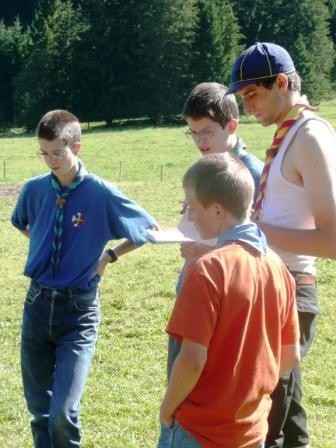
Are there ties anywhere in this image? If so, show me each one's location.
[231,136,248,153]
[50,161,86,280]
[253,94,316,222]
[216,222,268,256]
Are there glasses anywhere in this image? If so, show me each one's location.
[183,127,218,139]
[37,149,69,159]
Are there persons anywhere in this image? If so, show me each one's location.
[180,42,336,448]
[10,110,160,448]
[157,151,301,448]
[166,82,265,383]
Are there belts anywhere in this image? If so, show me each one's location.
[295,275,315,284]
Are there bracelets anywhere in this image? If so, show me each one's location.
[105,248,118,263]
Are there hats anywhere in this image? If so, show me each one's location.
[225,41,296,96]
